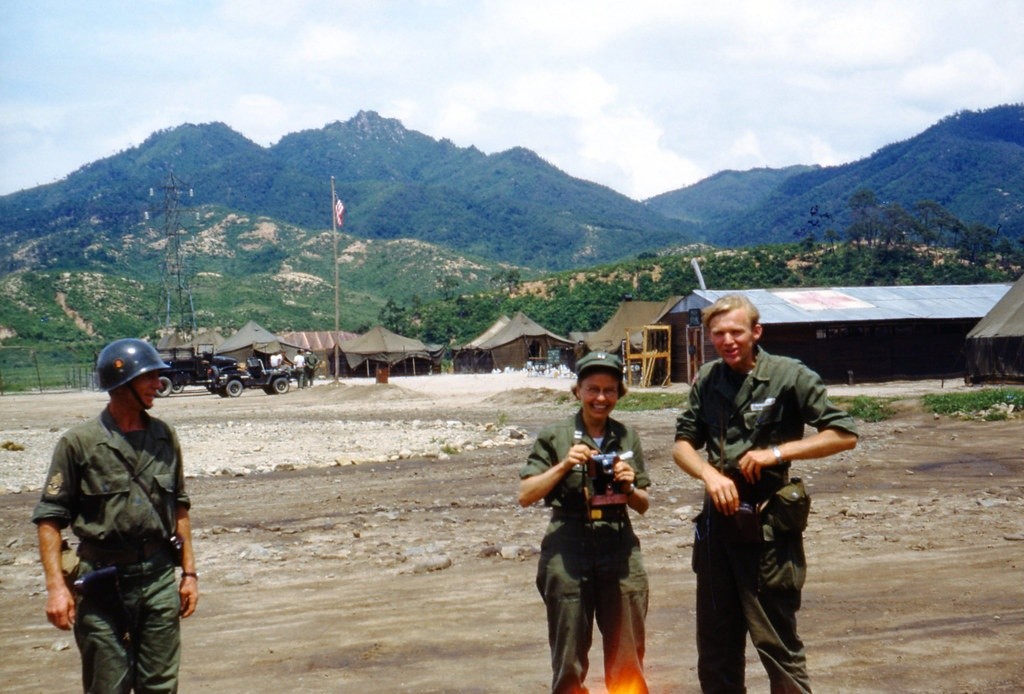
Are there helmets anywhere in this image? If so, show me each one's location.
[95,338,171,391]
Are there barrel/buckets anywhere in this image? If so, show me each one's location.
[376,368,388,383]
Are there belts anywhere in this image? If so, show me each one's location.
[552,508,627,520]
[75,541,163,563]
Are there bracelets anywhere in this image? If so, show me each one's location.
[627,484,634,496]
[182,572,197,579]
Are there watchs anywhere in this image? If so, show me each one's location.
[773,447,782,464]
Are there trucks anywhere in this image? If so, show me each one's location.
[140,343,241,394]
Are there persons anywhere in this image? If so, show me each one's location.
[269,351,292,383]
[294,349,320,389]
[674,294,859,693]
[30,338,200,694]
[520,350,652,694]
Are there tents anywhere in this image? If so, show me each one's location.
[216,320,310,369]
[967,273,1024,386]
[329,325,445,376]
[451,296,684,374]
[155,331,224,358]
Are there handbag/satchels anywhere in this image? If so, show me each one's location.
[691,515,716,578]
[774,476,811,534]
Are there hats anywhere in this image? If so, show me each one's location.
[574,351,624,377]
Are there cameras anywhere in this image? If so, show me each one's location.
[587,453,621,478]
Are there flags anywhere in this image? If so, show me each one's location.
[334,193,344,225]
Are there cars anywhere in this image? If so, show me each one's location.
[207,358,291,398]
